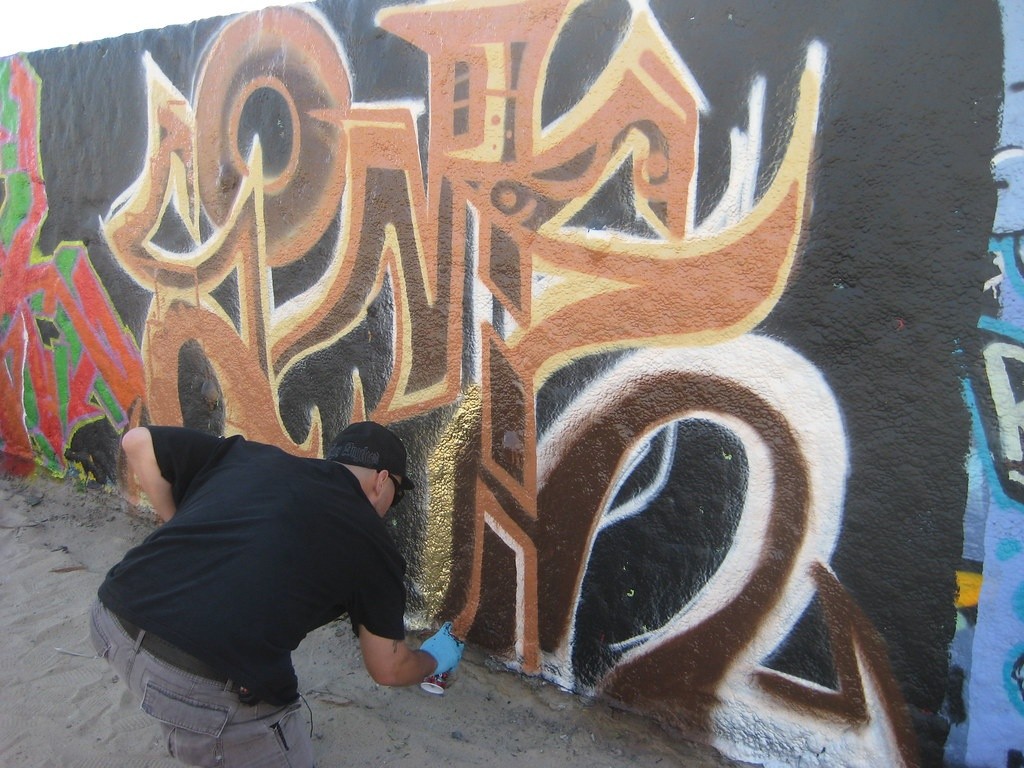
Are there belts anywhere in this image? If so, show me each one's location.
[117,615,237,685]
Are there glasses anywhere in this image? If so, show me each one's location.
[376,469,405,506]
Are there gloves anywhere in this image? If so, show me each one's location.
[420,622,464,675]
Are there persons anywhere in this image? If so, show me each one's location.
[86,421,465,768]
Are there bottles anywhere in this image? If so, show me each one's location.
[420,667,449,695]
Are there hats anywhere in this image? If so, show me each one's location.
[330,421,416,490]
